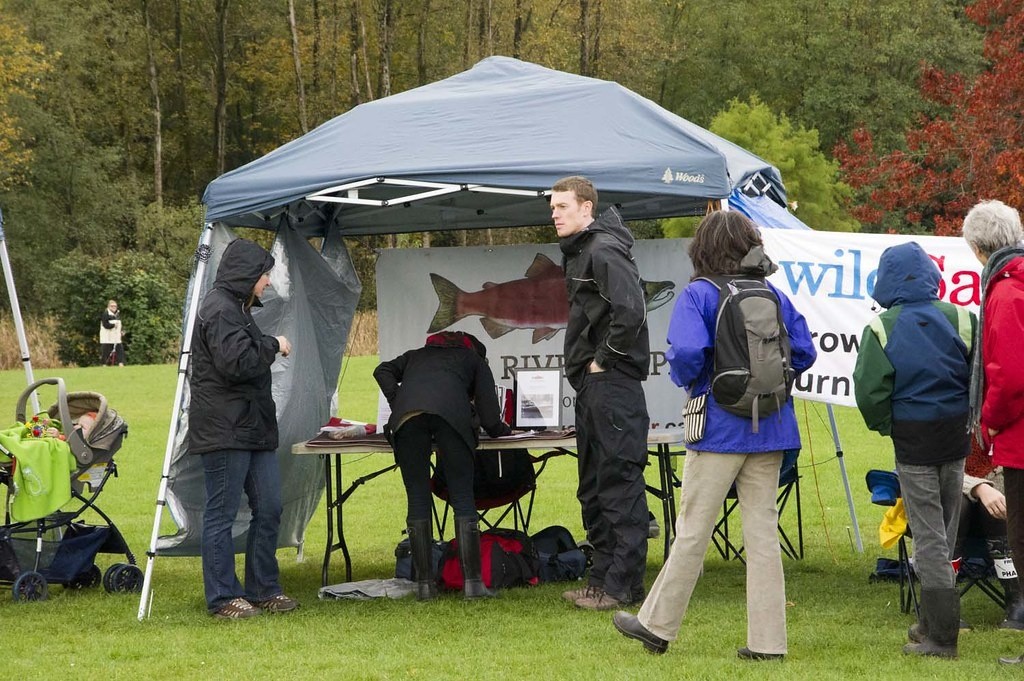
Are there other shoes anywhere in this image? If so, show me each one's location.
[613,611,668,654]
[736,645,785,664]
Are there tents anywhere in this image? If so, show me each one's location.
[136,56,862,620]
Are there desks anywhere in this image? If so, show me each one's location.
[292,430,685,587]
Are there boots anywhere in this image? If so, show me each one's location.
[909,623,926,643]
[406,518,442,601]
[904,588,961,661]
[454,515,495,600]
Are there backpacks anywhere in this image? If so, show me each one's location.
[693,273,796,434]
[530,526,587,584]
[446,528,538,592]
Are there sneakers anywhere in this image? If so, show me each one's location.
[575,590,622,610]
[577,539,595,568]
[999,617,1024,629]
[214,598,259,620]
[251,589,296,611]
[561,584,604,601]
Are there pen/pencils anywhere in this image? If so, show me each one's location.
[501,408,506,421]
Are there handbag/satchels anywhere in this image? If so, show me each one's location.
[397,535,442,581]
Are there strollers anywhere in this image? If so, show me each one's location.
[0,376,145,608]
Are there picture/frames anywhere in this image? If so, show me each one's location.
[512,367,563,430]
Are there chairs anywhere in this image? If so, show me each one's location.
[429,448,537,542]
[664,449,804,565]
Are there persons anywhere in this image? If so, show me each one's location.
[552,176,649,611]
[613,211,817,662]
[100,300,124,368]
[853,200,1024,661]
[373,331,510,601]
[46,411,98,447]
[187,239,302,618]
